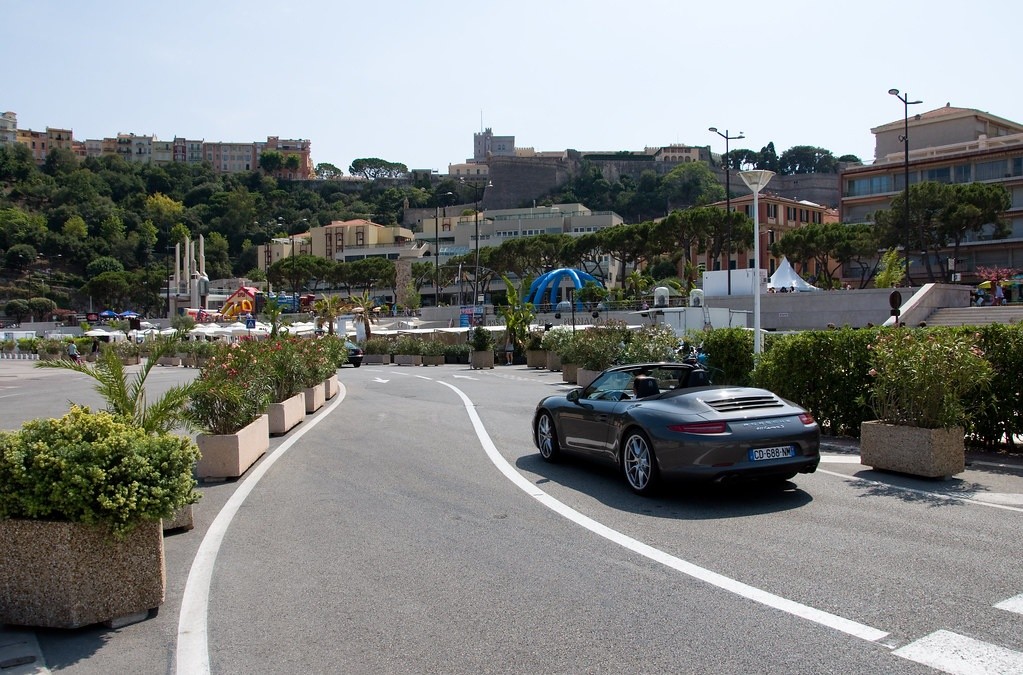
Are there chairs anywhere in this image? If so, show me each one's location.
[676,369,709,388]
[635,377,660,398]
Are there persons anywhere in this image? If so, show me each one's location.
[995,282,1004,306]
[766,282,900,294]
[827,321,927,330]
[392,304,417,317]
[586,301,650,312]
[672,338,708,364]
[630,374,647,399]
[66,340,80,361]
[505,333,515,365]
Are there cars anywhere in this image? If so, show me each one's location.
[336,340,365,367]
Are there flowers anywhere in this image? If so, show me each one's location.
[852,328,1000,435]
[524,322,678,370]
[186,332,350,434]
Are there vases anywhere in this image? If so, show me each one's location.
[325,374,338,399]
[577,367,600,385]
[562,363,583,384]
[546,350,563,373]
[266,392,306,434]
[195,413,270,478]
[859,419,964,477]
[527,350,547,370]
[303,382,326,412]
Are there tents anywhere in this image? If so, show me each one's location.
[767,256,823,292]
[83,320,376,343]
[99,310,140,323]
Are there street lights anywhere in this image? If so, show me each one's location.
[419,187,453,306]
[252,220,284,295]
[707,127,746,294]
[885,86,923,286]
[165,245,179,312]
[38,252,63,323]
[277,217,307,314]
[459,180,495,304]
[19,254,41,316]
[734,165,777,370]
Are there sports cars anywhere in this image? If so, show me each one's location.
[531,361,822,500]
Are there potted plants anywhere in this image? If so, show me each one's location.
[0,317,246,628]
[361,326,497,370]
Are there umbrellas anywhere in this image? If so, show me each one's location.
[979,278,1013,289]
